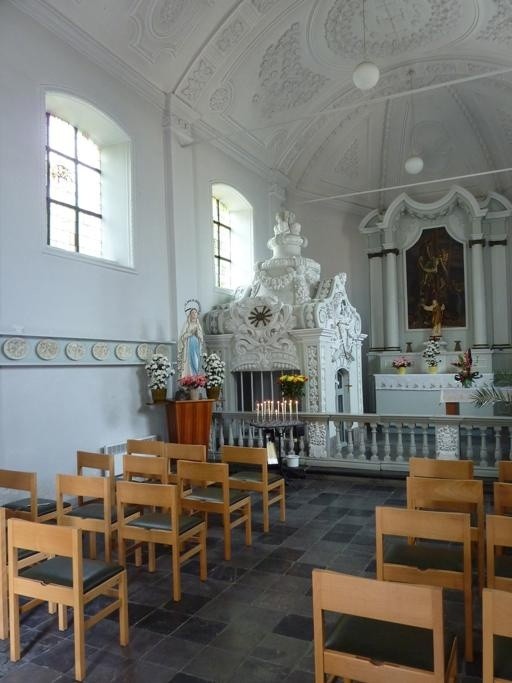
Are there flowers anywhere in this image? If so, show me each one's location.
[392,356,412,370]
[200,351,225,388]
[423,341,441,367]
[178,374,208,390]
[146,353,176,391]
[277,375,308,403]
[451,349,483,383]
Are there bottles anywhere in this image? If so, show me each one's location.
[454,340,462,351]
[406,342,413,352]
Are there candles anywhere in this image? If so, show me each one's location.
[256,400,301,423]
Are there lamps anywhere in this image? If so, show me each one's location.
[406,68,423,175]
[353,0,379,90]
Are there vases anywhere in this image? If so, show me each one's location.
[204,385,221,400]
[287,454,299,468]
[394,365,408,374]
[187,387,199,400]
[149,387,168,401]
[460,377,473,389]
[427,364,439,374]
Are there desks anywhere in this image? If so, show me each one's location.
[250,422,307,485]
[154,399,225,463]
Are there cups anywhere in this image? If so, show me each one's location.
[286,454,300,468]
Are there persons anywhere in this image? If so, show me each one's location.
[176,308,204,378]
[421,300,445,336]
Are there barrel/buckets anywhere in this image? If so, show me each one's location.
[286,455,299,468]
